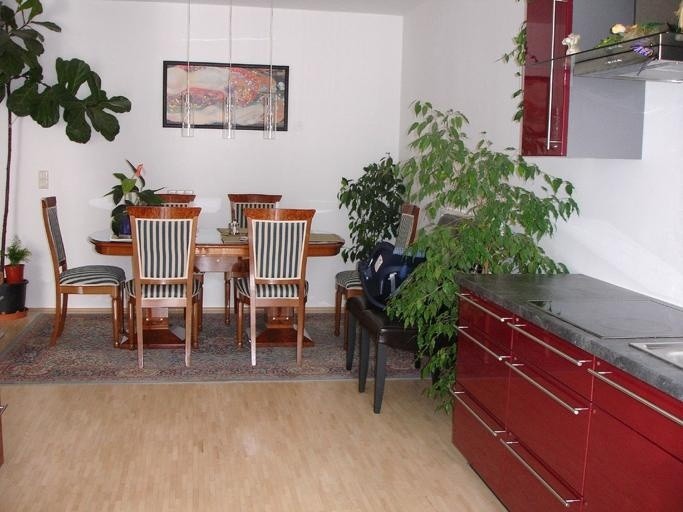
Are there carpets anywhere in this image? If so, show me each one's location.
[0,312,456,385]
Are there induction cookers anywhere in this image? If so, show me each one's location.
[524,297,683,340]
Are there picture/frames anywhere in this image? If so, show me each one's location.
[163,60,288,131]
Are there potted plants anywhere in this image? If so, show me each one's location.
[0,0,131,320]
[103,159,162,239]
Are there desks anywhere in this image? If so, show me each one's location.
[86,228,345,350]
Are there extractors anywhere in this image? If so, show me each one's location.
[570,32,683,86]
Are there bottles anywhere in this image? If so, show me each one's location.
[228,218,239,236]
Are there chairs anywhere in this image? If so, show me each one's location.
[224,193,283,326]
[159,193,197,208]
[125,205,203,369]
[42,195,127,347]
[344,212,483,414]
[334,204,419,339]
[233,206,316,366]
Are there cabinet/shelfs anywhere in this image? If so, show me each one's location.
[520,0,645,159]
[0,404,9,469]
[451,290,683,512]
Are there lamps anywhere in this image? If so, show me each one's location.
[180,0,278,142]
